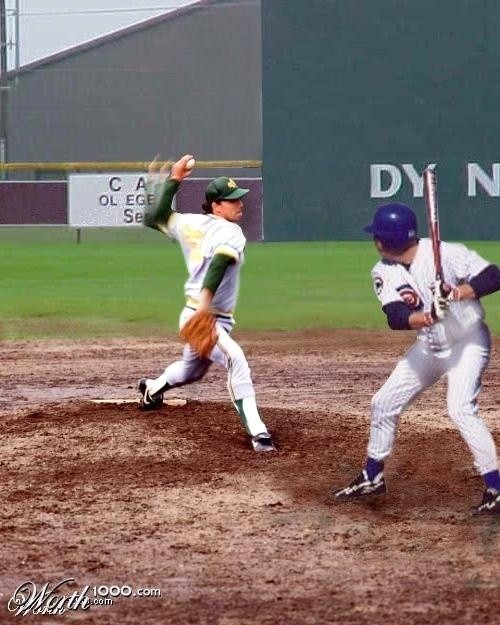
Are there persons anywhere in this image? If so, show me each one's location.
[331,200,499,514]
[136,154,281,457]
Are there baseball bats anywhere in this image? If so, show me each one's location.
[423,169,447,307]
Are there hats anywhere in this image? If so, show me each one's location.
[205,177,251,203]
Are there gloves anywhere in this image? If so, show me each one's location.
[424,279,460,327]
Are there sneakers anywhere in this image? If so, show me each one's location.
[326,468,387,502]
[469,489,500,515]
[139,377,164,409]
[251,431,276,453]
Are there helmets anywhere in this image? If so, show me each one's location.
[364,204,418,251]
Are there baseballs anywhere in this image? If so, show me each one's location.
[185,159,195,169]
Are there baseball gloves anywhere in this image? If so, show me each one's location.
[180,312,218,357]
